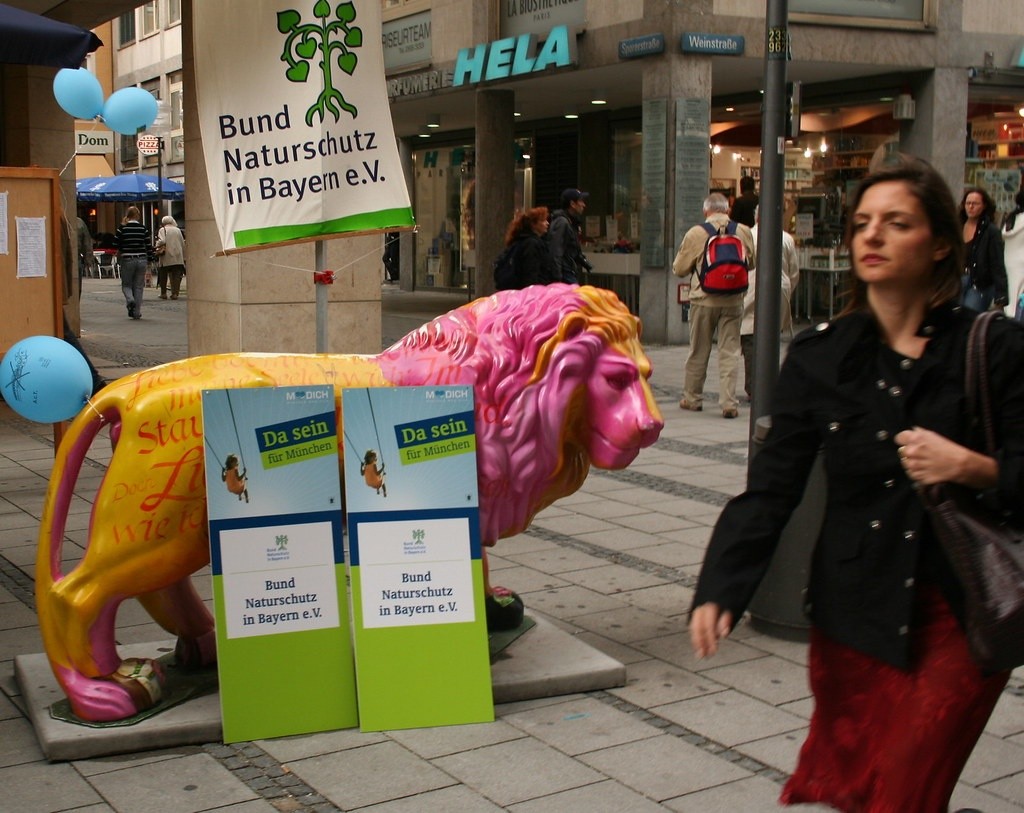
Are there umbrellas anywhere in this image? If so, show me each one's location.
[75,171,184,203]
[0,3,104,71]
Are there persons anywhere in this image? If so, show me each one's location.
[155,216,185,300]
[957,187,1010,312]
[671,192,756,418]
[77,216,93,300]
[729,175,759,227]
[740,205,800,403]
[685,152,1024,813]
[548,188,594,285]
[506,207,558,284]
[112,207,152,320]
[1001,192,1024,318]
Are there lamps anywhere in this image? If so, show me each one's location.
[427,114,440,128]
[418,124,431,137]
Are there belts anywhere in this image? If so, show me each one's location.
[120,254,147,258]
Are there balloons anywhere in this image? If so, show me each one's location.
[54,67,104,119]
[0,335,93,424]
[102,88,159,136]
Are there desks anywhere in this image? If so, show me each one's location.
[85,249,118,278]
[793,264,853,323]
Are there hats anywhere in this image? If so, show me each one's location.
[561,188,590,203]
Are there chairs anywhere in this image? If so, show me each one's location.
[80,252,120,279]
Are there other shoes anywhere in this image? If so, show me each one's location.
[170,293,178,299]
[723,408,739,418]
[133,313,141,319]
[128,302,136,316]
[159,293,167,299]
[746,394,751,401]
[680,400,703,411]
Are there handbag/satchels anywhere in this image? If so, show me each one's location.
[155,226,166,256]
[911,309,1024,673]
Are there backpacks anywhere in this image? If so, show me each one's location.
[698,221,750,295]
[493,237,525,290]
